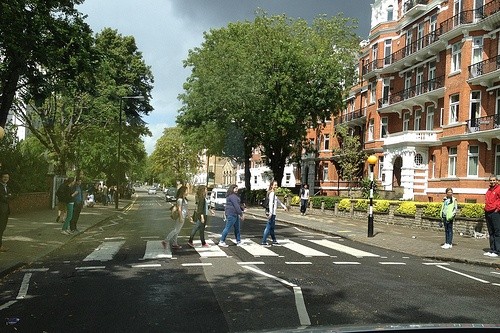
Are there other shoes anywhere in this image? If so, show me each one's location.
[484,252,498,257]
[187,241,196,247]
[237,242,245,245]
[160,240,168,250]
[262,242,269,245]
[272,242,281,246]
[218,242,229,247]
[440,243,453,248]
[61,229,79,235]
[172,245,182,248]
[201,243,212,247]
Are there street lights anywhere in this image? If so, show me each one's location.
[114,96,145,208]
[366,154,379,237]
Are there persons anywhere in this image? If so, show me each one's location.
[0,173,14,247]
[218,184,244,247]
[300,184,309,216]
[262,180,287,244]
[440,187,457,249]
[56,177,84,235]
[483,175,500,256]
[84,183,129,208]
[161,186,189,249]
[187,185,210,247]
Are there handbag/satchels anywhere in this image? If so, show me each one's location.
[170,205,179,219]
[262,198,269,208]
[193,211,197,222]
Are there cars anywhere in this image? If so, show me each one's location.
[165,188,177,202]
[206,188,227,210]
[148,187,157,195]
